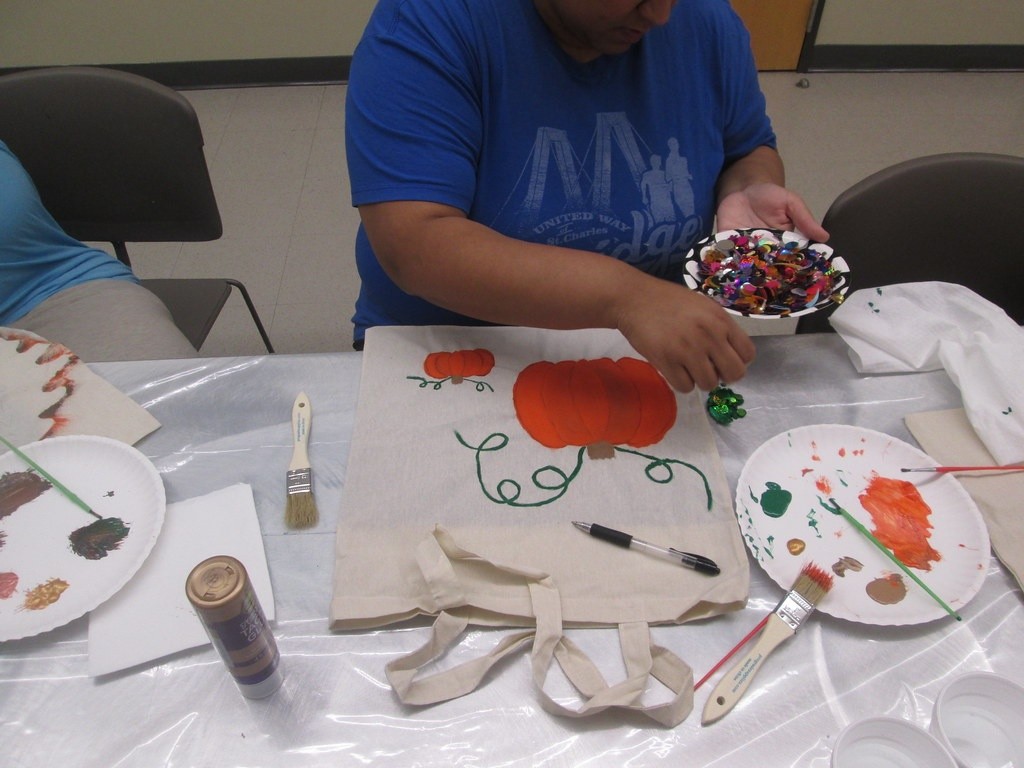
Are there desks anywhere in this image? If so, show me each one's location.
[0,348,1024,768]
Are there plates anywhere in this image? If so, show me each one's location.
[735,423,990,626]
[0,435,167,642]
[683,227,852,319]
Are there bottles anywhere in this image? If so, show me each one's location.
[185,555,286,700]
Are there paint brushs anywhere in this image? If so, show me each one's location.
[830,498,962,621]
[0,436,102,519]
[901,465,1024,472]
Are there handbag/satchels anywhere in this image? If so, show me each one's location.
[328,326,750,727]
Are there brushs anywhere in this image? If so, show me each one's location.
[701,560,833,726]
[285,391,318,529]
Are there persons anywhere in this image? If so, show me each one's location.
[0,138,201,360]
[346,0,829,391]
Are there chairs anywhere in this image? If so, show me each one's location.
[0,72,275,356]
[799,154,1023,331]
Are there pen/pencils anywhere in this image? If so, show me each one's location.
[572,520,720,574]
